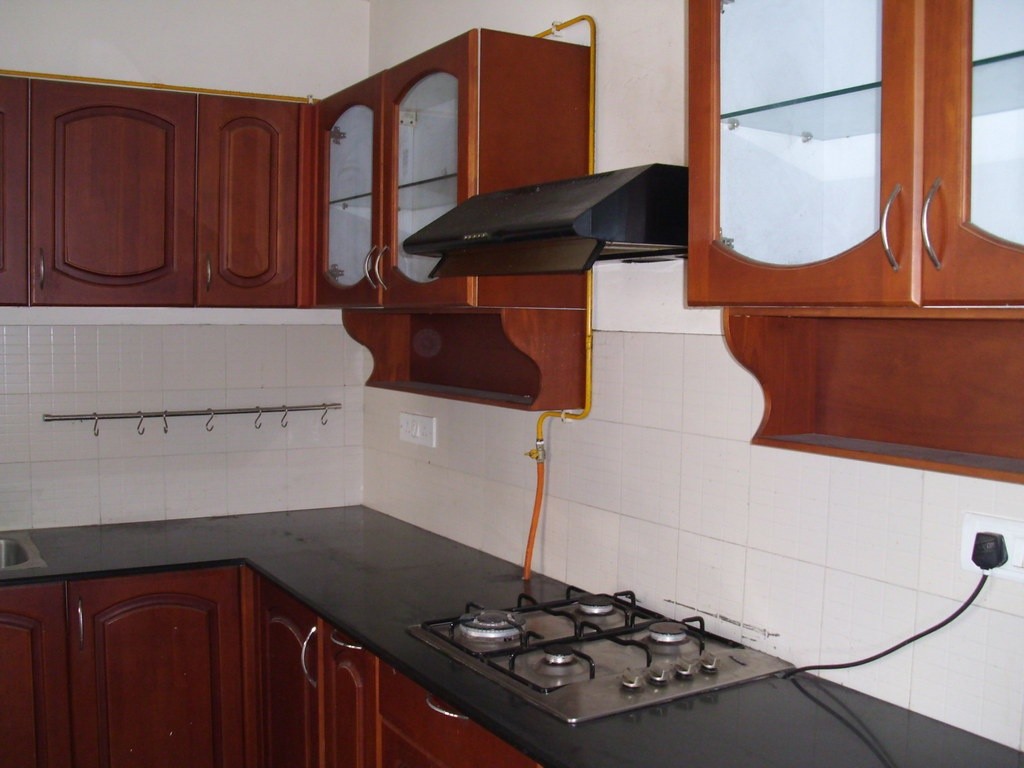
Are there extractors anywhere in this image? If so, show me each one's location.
[403,164,689,277]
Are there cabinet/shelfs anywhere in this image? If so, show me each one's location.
[0,564,544,768]
[959,511,1024,584]
[684,0,1024,488]
[1,29,592,411]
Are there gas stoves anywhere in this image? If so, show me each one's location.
[404,587,796,724]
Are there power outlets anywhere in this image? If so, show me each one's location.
[399,412,436,448]
